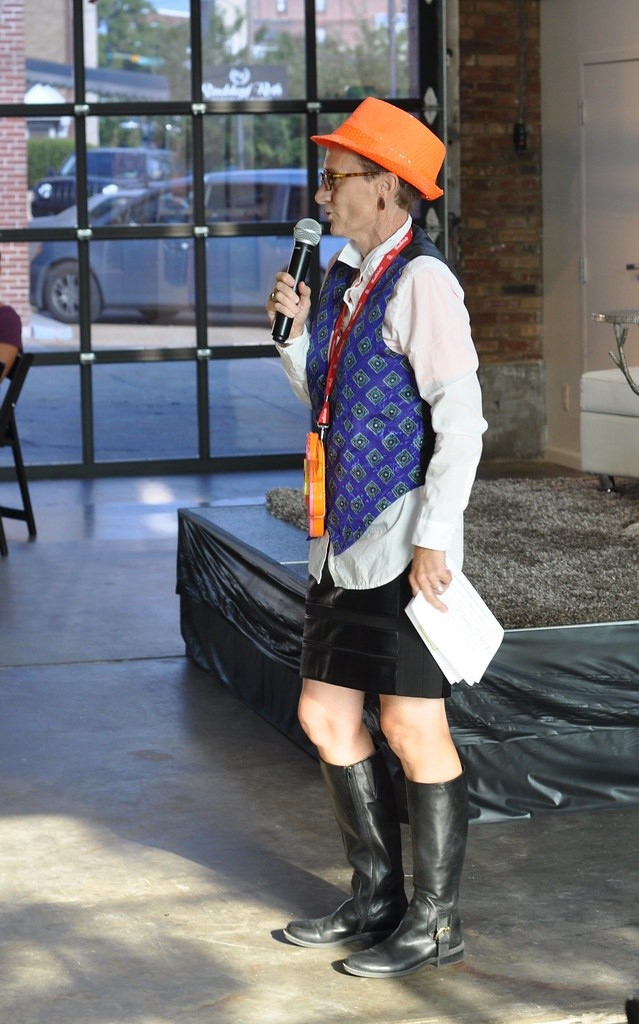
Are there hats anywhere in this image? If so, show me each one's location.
[309,97,447,202]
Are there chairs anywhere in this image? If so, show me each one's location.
[0,352,36,559]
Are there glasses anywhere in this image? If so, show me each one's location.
[319,167,381,192]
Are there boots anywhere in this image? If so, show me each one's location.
[284,749,410,947]
[343,762,469,979]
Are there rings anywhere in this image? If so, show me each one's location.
[270,291,277,304]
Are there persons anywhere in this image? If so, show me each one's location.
[267,97,505,978]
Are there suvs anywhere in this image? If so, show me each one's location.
[30,168,353,326]
[30,147,188,217]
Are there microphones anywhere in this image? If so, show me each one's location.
[270,218,322,343]
